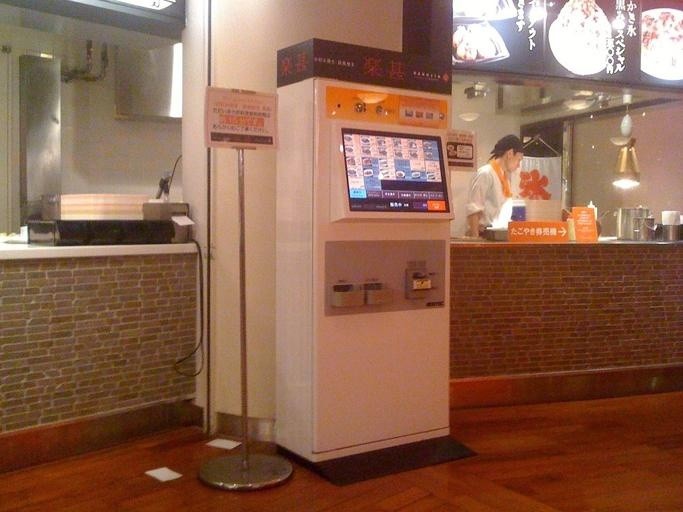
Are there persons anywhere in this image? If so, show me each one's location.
[463,133,525,239]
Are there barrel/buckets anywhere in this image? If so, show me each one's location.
[633,217,656,242]
[617,207,648,240]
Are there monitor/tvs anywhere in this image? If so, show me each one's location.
[340,126,452,215]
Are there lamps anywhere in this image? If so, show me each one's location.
[609,92,642,190]
[563,89,595,111]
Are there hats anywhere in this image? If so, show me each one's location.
[491,134,524,154]
